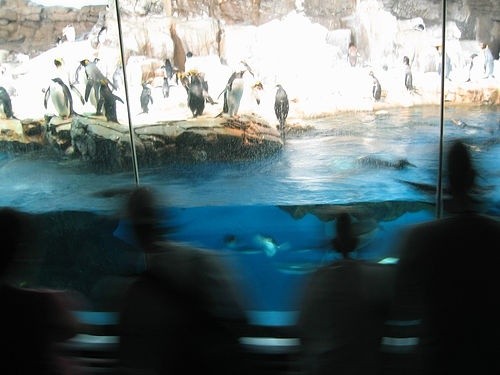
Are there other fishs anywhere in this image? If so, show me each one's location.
[194,222,400,281]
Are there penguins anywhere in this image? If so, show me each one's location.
[479,43,496,79]
[465,54,480,81]
[353,154,421,171]
[368,72,380,102]
[403,56,414,94]
[215,68,248,118]
[44,78,78,119]
[275,83,289,129]
[53,57,219,117]
[451,118,487,131]
[412,23,457,82]
[345,42,361,70]
[97,79,124,125]
[0,85,19,120]
